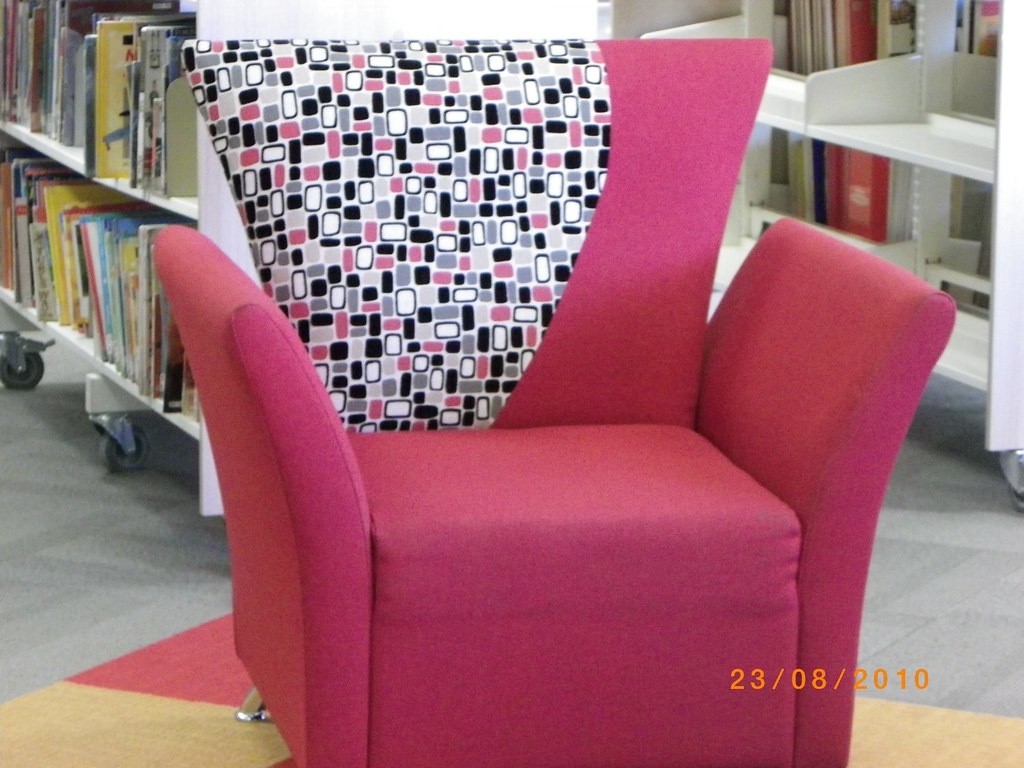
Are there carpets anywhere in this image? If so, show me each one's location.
[0,610,1024,768]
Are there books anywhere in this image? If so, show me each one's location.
[0,0,206,423]
[784,0,1004,247]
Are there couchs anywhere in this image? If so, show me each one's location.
[156,39,957,768]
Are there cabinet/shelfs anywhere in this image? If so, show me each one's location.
[598,0,1024,515]
[0,0,599,519]
